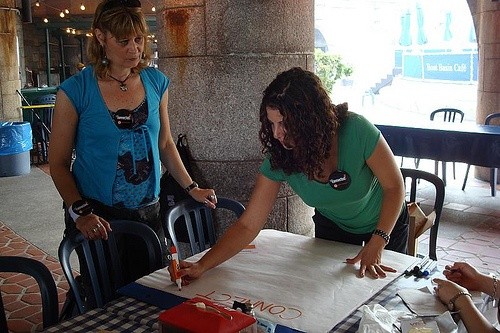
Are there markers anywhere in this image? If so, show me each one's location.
[170,246,183,291]
[404,256,439,278]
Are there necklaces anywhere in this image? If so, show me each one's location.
[106,73,132,92]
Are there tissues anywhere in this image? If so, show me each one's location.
[156,297,257,333]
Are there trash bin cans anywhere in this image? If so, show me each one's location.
[0,121,31,177]
[21,87,58,143]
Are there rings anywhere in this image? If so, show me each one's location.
[93,229,97,232]
[89,232,92,235]
[210,195,216,199]
[205,200,209,204]
[97,222,103,227]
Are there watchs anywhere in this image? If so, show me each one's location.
[449,291,472,311]
[185,181,199,192]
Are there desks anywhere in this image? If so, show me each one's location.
[44,229,499,333]
[374,118,500,170]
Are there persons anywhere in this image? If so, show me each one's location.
[167,66,408,287]
[75,63,86,72]
[433,262,500,333]
[49,0,218,308]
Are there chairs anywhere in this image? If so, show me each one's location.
[0,255,59,333]
[412,108,465,186]
[399,168,444,261]
[460,113,500,196]
[167,197,247,261]
[58,219,162,316]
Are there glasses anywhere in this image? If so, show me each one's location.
[96,0,141,28]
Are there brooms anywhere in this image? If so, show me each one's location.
[16,89,76,161]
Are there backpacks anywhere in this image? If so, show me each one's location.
[159,135,216,243]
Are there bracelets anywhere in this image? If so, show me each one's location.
[373,229,390,244]
[491,276,497,300]
[68,201,94,221]
